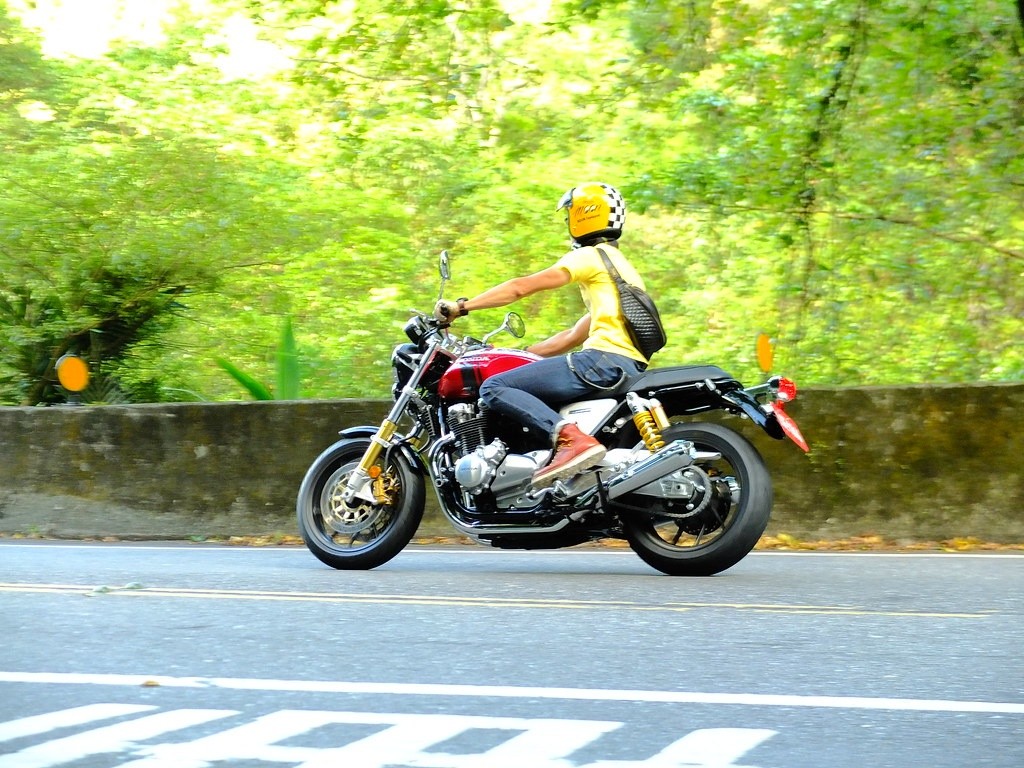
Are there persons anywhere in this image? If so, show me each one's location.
[434,182,650,489]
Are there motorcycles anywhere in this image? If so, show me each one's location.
[295,250,811,576]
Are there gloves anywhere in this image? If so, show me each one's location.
[432,299,460,325]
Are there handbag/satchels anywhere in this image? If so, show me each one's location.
[597,246,666,362]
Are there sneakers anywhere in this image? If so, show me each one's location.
[531,423,606,488]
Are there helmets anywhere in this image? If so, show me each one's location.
[564,182,626,239]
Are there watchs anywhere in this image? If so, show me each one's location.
[456,297,468,316]
